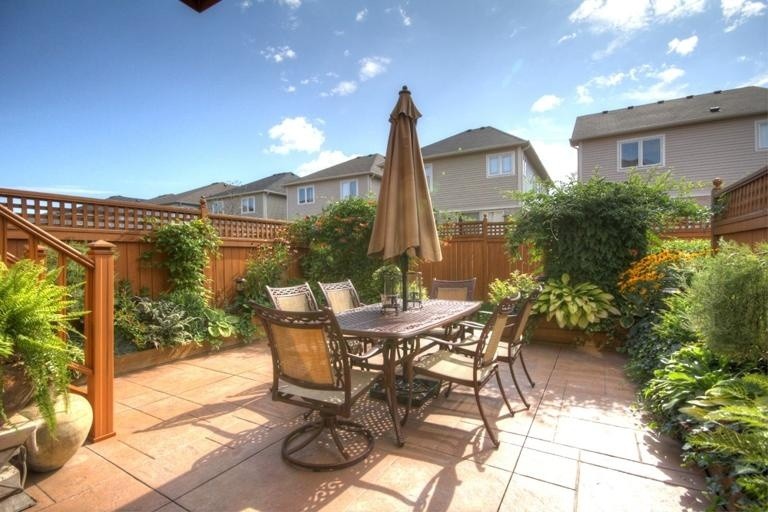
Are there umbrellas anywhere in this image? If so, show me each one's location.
[366,87,443,380]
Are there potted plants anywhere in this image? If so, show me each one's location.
[371,263,401,302]
[0,260,92,425]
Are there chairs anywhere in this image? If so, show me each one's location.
[444,285,543,408]
[265,278,476,393]
[399,292,516,448]
[247,299,385,468]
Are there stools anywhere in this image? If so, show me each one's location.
[334,298,482,408]
